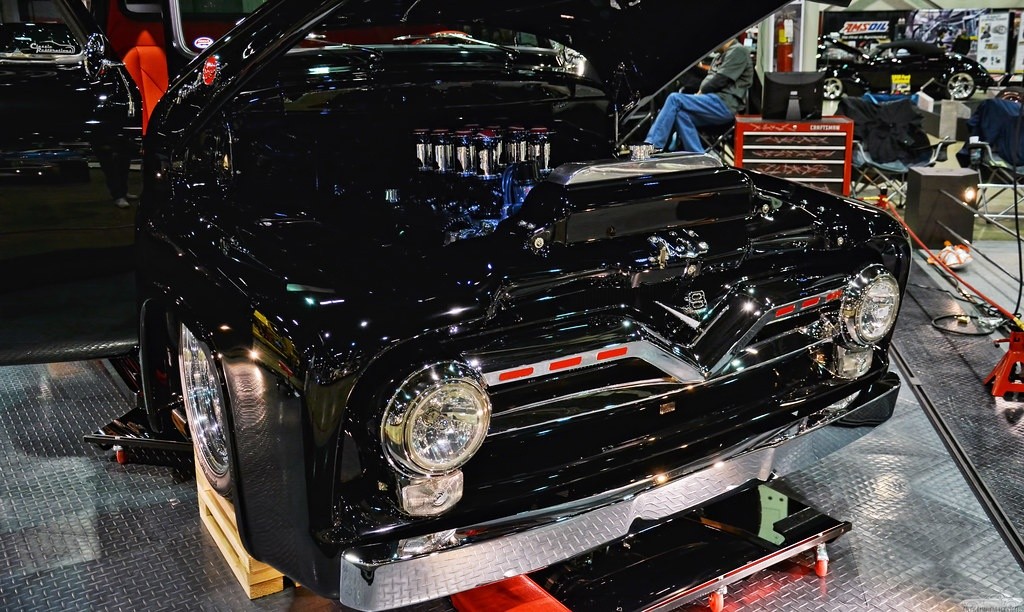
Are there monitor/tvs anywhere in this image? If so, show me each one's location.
[762,71,825,121]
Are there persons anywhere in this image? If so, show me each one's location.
[644,39,753,154]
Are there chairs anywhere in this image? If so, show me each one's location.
[845,92,957,210]
[960,98,1024,223]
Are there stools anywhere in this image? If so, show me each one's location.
[693,120,737,169]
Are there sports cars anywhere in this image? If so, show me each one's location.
[813,33,1000,103]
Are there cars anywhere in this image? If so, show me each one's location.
[1,0,142,203]
[120,0,910,611]
